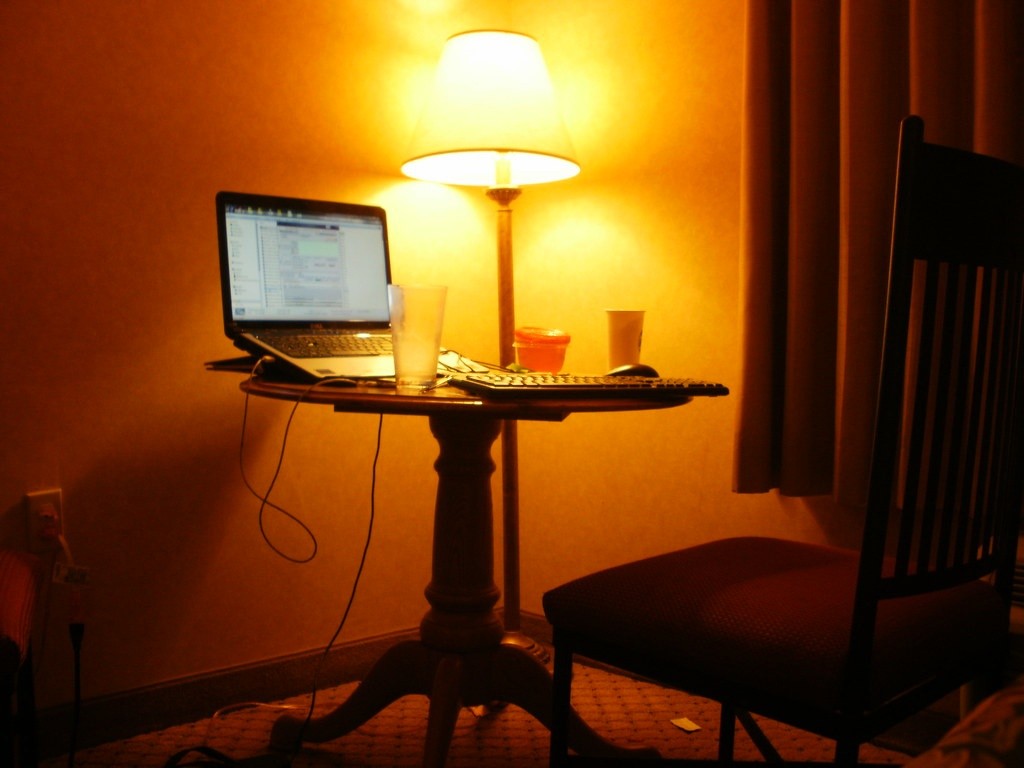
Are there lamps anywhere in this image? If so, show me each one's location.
[395,28,581,666]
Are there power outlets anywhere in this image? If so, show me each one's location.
[26,490,65,555]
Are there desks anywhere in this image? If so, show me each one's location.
[240,375,694,768]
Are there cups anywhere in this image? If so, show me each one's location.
[606,310,646,370]
[387,284,448,389]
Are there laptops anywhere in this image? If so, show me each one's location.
[215,191,490,386]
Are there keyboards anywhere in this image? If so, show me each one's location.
[446,372,729,401]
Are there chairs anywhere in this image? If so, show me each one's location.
[542,113,1024,768]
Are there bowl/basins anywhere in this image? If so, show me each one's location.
[512,328,570,375]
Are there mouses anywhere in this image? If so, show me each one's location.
[606,364,659,376]
